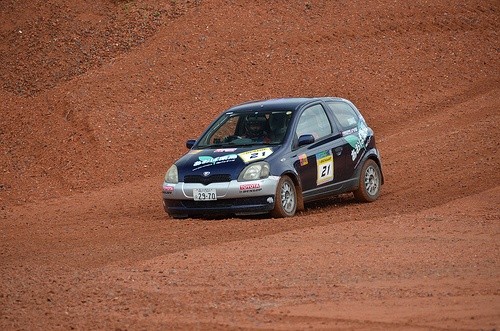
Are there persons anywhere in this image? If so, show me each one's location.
[212,113,270,144]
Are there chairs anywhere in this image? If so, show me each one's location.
[268,112,288,141]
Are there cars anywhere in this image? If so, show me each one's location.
[163,96,384,220]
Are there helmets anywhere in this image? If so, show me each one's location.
[245,113,268,136]
